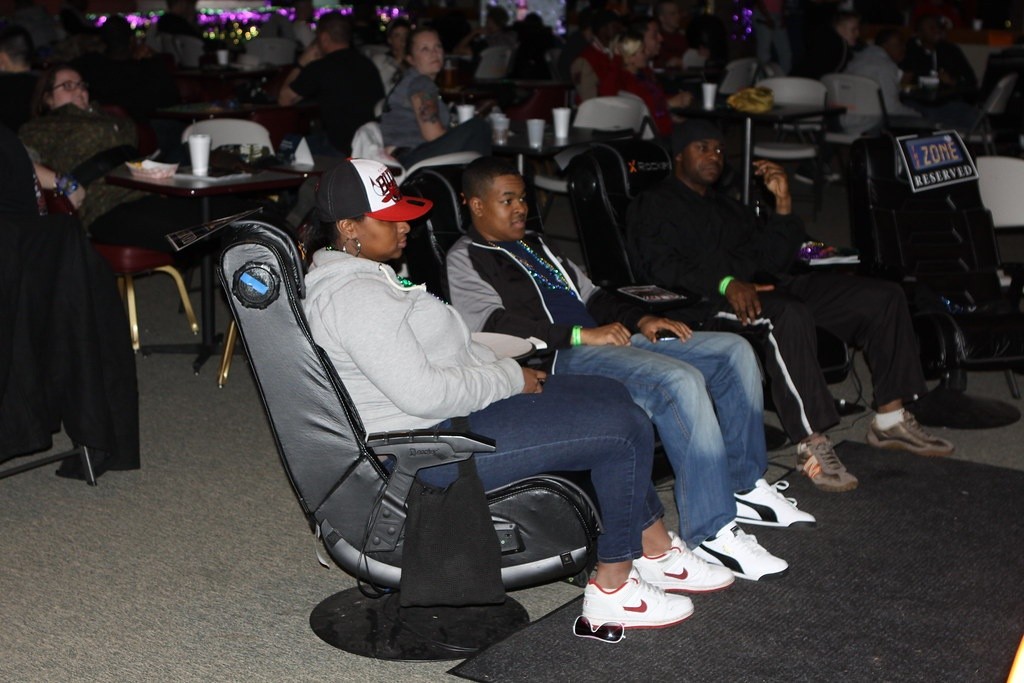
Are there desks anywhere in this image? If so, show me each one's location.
[502,80,572,119]
[492,129,635,157]
[671,103,847,207]
[179,66,283,99]
[262,154,345,177]
[151,100,321,150]
[106,162,304,375]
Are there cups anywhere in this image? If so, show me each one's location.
[218,49,228,65]
[554,108,570,146]
[490,113,511,145]
[188,134,211,176]
[456,104,475,125]
[527,119,548,148]
[702,83,717,109]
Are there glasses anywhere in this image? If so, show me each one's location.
[573,616,627,644]
[48,81,88,93]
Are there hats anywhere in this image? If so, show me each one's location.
[316,157,434,224]
[670,119,725,157]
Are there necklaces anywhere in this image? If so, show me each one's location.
[485,236,577,298]
[326,245,450,305]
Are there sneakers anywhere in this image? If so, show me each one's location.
[734,478,817,529]
[581,566,696,629]
[867,410,955,457]
[798,434,858,492]
[632,536,735,592]
[682,520,790,581]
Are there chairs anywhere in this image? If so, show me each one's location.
[0,4,1024,661]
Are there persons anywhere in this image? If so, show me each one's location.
[19,68,245,268]
[0,0,1024,169]
[448,157,817,582]
[299,157,737,630]
[628,116,955,492]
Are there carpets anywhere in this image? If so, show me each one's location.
[446,440,1023,683]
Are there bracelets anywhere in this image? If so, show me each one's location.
[718,275,734,296]
[293,59,304,70]
[54,172,78,198]
[573,325,583,346]
